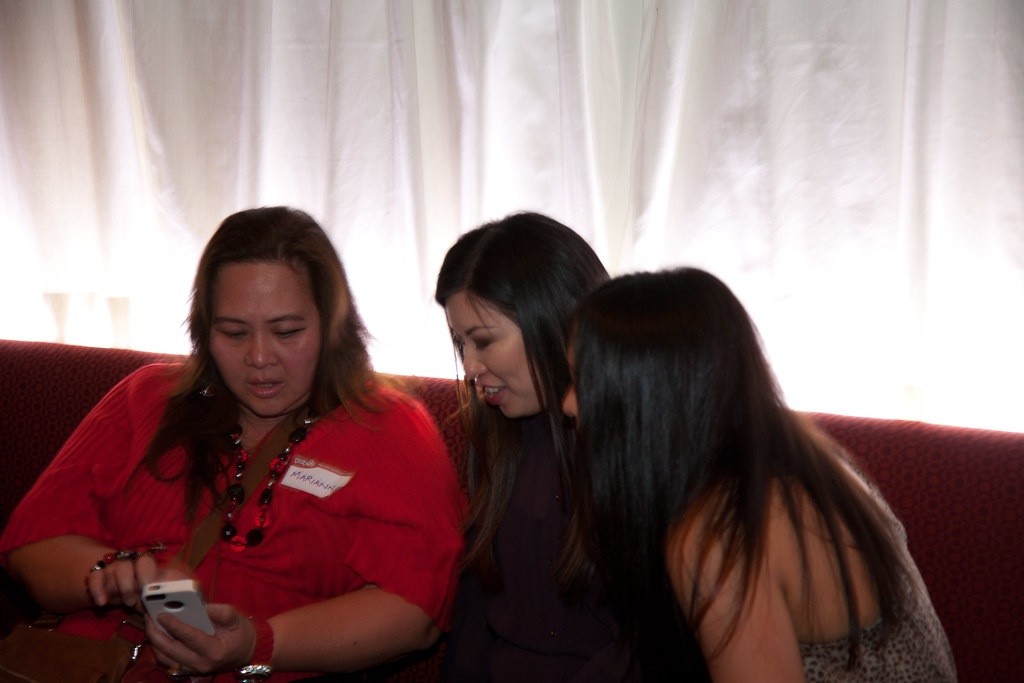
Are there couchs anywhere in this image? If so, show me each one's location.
[2,339,1024,683]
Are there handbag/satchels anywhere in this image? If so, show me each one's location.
[0,624,130,683]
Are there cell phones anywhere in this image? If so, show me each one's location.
[142,578,214,642]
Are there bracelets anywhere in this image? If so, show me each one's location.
[84,546,154,609]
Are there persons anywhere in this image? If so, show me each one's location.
[561,266,958,683]
[398,212,714,683]
[1,205,470,683]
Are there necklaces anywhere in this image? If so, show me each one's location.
[213,381,335,549]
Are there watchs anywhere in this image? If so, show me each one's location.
[232,612,277,683]
